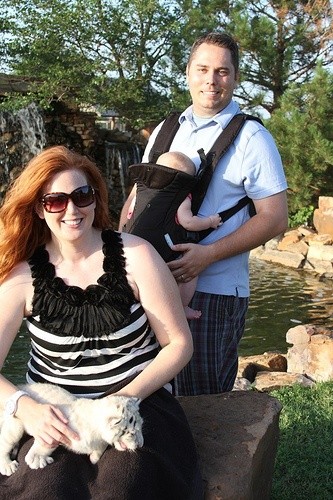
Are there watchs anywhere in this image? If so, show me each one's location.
[4,391,28,419]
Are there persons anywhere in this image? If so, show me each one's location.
[0,143,195,500]
[116,32,289,397]
[127,152,224,319]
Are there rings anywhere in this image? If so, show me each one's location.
[180,275,184,281]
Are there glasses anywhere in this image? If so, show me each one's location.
[38,185,96,213]
